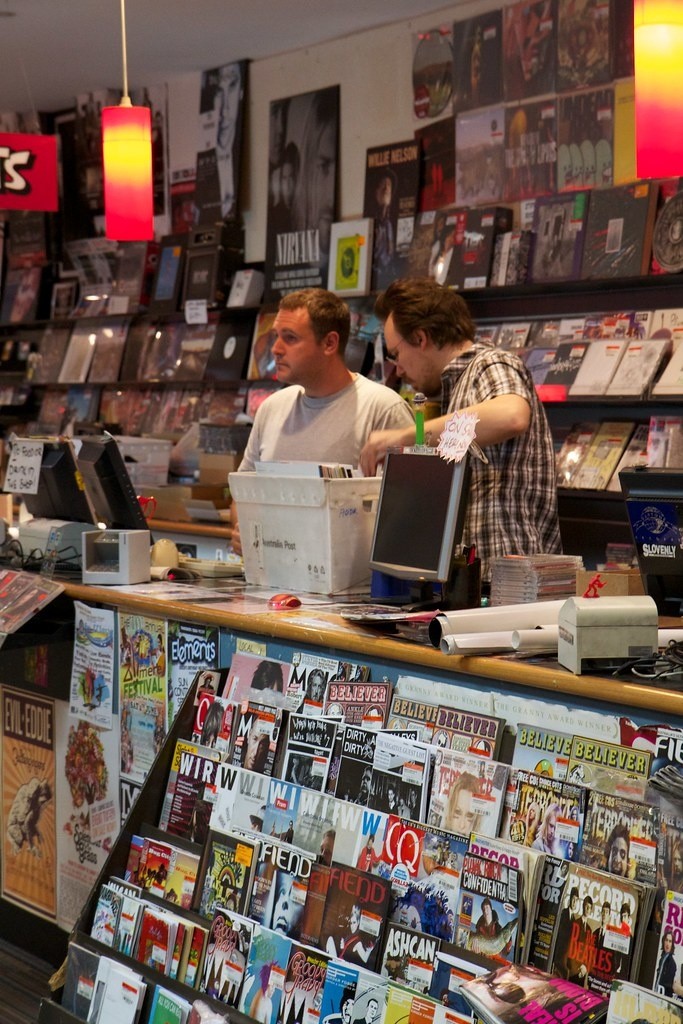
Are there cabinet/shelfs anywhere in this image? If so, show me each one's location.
[0,281,682,618]
[37,664,683,1024]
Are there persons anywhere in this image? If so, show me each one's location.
[567,896,594,988]
[250,806,265,832]
[447,771,484,839]
[609,904,634,980]
[654,931,678,997]
[568,807,579,821]
[199,62,243,224]
[585,902,613,993]
[284,821,295,843]
[270,857,308,942]
[601,825,631,881]
[230,286,419,556]
[531,803,564,855]
[297,668,326,719]
[347,769,420,823]
[319,830,335,868]
[357,835,380,873]
[366,163,401,286]
[553,886,580,978]
[352,694,683,999]
[468,897,512,953]
[244,716,271,773]
[523,802,541,847]
[269,89,339,286]
[360,276,564,598]
[564,843,577,861]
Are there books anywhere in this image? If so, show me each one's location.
[0,177,683,490]
[52,873,682,1024]
[124,648,394,974]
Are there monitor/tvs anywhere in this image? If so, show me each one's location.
[8,437,95,526]
[338,446,472,609]
[556,487,636,571]
[619,466,683,617]
[76,435,155,547]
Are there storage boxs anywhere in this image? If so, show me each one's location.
[227,471,383,594]
[198,454,241,485]
[140,486,227,522]
[117,437,173,489]
[199,424,252,454]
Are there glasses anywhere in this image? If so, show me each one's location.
[386,339,405,365]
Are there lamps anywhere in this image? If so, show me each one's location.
[101,0,156,244]
[630,0,683,180]
